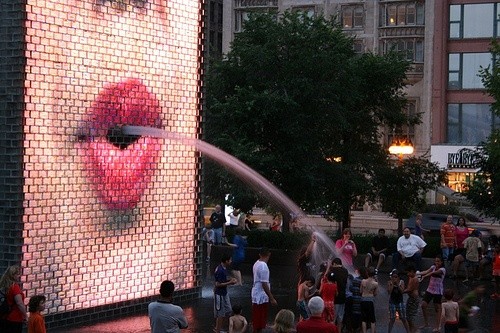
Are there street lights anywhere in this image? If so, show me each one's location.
[389,136,413,237]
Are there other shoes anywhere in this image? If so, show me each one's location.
[206,257,210,263]
[462,279,469,282]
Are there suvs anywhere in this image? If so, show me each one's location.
[405,213,493,241]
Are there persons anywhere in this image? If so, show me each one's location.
[359,267,378,333]
[251,247,278,333]
[224,229,245,287]
[209,205,227,254]
[27,295,46,333]
[0,265,28,333]
[229,305,248,333]
[228,209,241,244]
[364,229,391,279]
[238,213,255,231]
[202,223,214,262]
[213,255,238,333]
[388,214,500,333]
[264,309,296,333]
[291,218,300,231]
[270,214,282,232]
[296,228,362,333]
[148,280,188,333]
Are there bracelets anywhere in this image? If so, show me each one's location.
[312,240,315,242]
